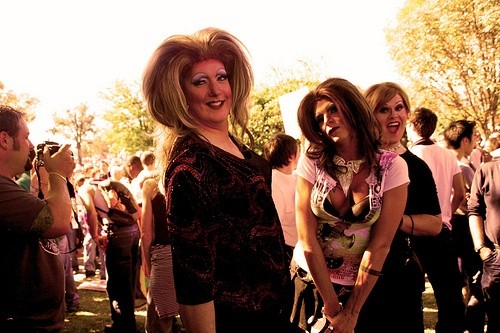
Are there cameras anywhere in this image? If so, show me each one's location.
[36,141,73,162]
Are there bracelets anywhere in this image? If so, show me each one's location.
[48,171,68,183]
[321,302,343,318]
[398,216,403,229]
[407,214,419,235]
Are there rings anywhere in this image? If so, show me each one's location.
[327,325,334,331]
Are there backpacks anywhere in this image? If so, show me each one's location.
[85,176,141,227]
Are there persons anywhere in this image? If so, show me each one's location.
[15,141,184,333]
[142,27,295,333]
[0,104,77,333]
[296,78,409,333]
[355,82,442,333]
[263,107,500,333]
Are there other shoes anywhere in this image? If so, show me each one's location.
[67,305,79,312]
[104,324,137,333]
